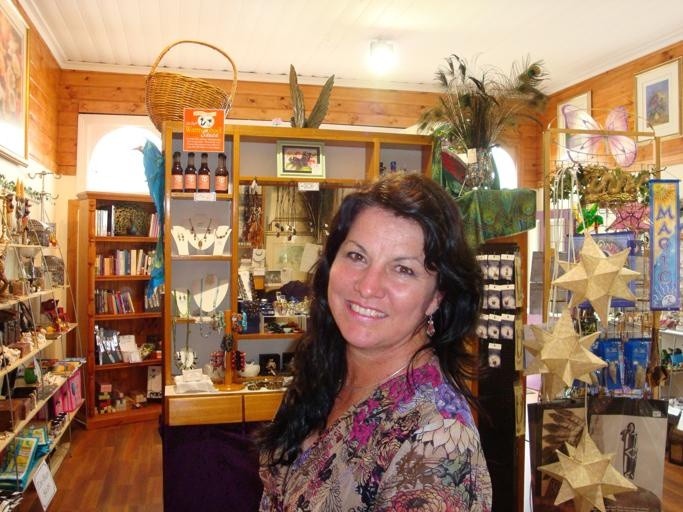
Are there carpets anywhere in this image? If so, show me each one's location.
[159,413,272,512]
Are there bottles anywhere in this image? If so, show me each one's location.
[183,152,198,194]
[197,153,210,193]
[214,153,228,194]
[171,151,183,193]
[390,161,396,173]
[379,162,384,176]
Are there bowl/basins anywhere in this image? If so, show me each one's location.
[237,364,260,377]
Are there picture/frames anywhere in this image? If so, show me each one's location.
[556,90,593,166]
[277,140,326,179]
[634,56,683,147]
[0,0,30,168]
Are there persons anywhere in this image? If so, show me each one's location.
[259,170,493,512]
[621,422,638,479]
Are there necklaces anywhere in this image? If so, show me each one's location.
[171,177,334,389]
[341,349,427,394]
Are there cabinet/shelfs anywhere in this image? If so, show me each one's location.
[162,121,441,426]
[74,191,165,431]
[0,186,87,512]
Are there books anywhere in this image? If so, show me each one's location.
[95,202,160,365]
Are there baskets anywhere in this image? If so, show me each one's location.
[145,39,237,131]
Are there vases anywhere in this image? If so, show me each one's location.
[465,147,495,190]
